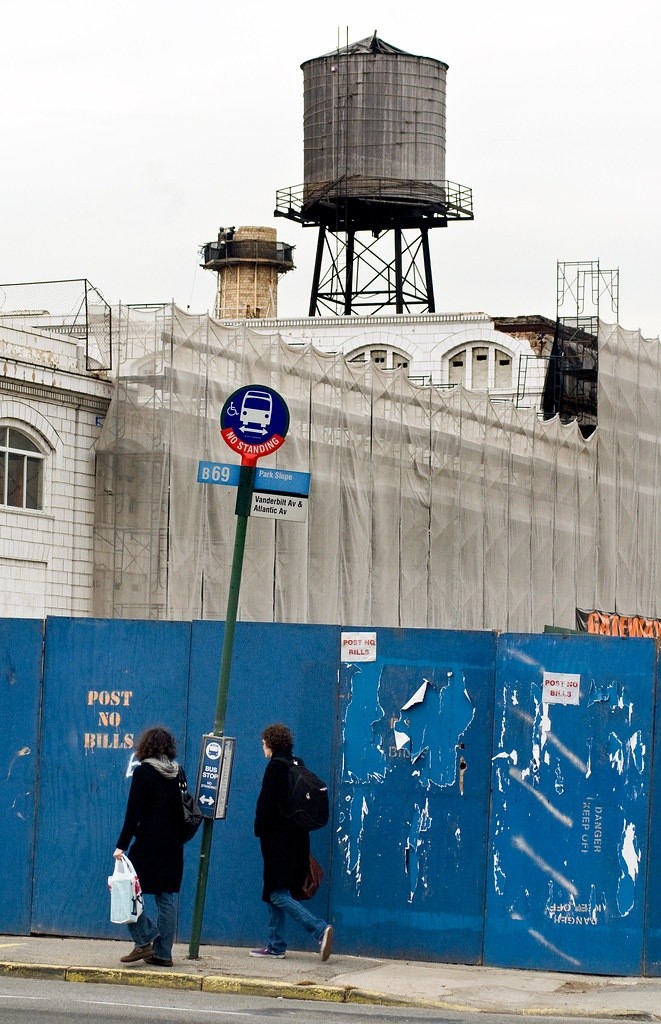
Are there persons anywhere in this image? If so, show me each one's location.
[113,727,188,967]
[249,725,333,962]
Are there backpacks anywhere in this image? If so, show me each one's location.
[273,756,329,830]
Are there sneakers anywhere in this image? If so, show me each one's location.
[250,946,285,959]
[319,926,334,961]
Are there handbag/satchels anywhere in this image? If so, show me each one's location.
[177,767,203,842]
[108,854,144,924]
[289,851,323,900]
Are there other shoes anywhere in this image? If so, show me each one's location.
[143,954,173,967]
[120,944,155,962]
